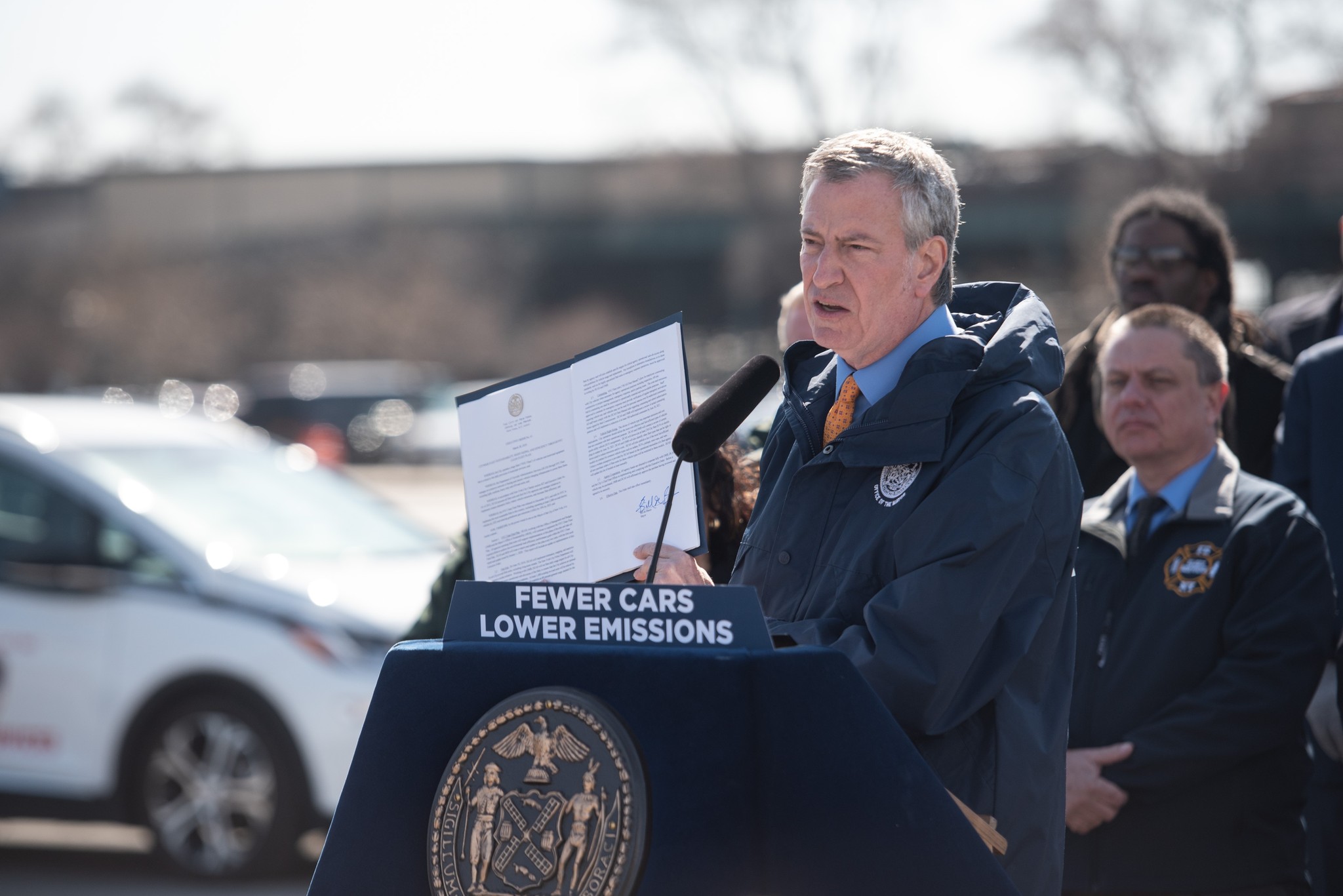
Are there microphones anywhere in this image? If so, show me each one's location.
[642,355,782,585]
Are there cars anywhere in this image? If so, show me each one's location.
[0,391,462,879]
[235,355,431,468]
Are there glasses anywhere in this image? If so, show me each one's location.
[1112,245,1205,273]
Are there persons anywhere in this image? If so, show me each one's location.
[633,129,1084,896]
[403,192,1342,896]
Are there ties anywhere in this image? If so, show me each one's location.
[1128,497,1164,558]
[823,375,860,448]
